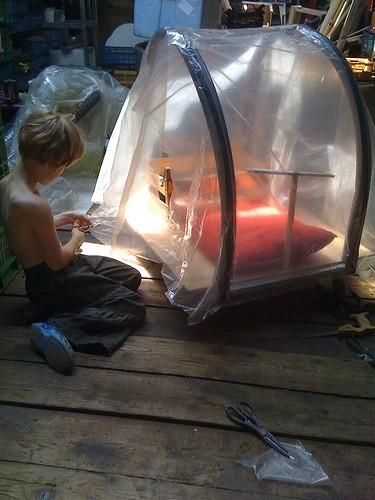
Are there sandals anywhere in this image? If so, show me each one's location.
[29,322,74,375]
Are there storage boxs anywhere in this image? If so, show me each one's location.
[0,1,111,179]
[360,11,375,55]
[133,0,203,39]
[201,0,233,29]
[146,146,271,228]
[113,70,138,91]
[98,43,139,71]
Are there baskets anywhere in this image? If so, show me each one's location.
[98,36,137,90]
[0,0,50,91]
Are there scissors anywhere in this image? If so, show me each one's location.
[73,222,100,236]
[225,401,290,458]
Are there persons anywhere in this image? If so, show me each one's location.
[0,110,145,375]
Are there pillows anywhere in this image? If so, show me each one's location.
[185,206,338,276]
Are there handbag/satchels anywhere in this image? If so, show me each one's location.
[223,3,265,28]
